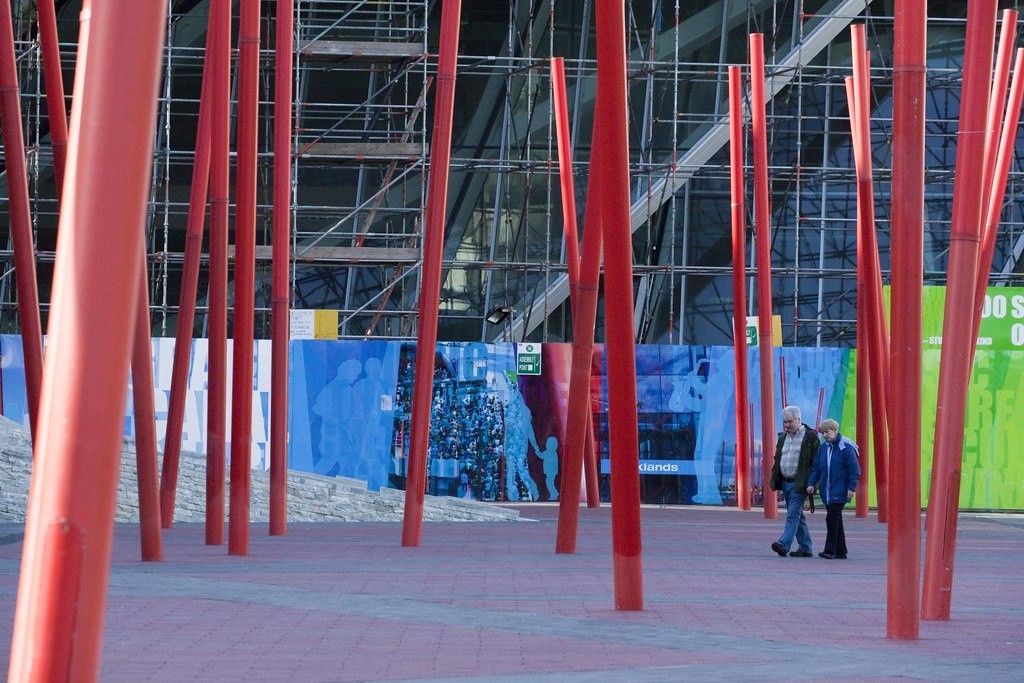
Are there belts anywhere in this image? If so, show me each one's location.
[783,477,796,483]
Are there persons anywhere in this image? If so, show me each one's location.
[807,418,861,560]
[769,402,820,557]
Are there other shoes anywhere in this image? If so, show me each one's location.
[789,550,813,557]
[819,551,848,559]
[771,542,788,556]
